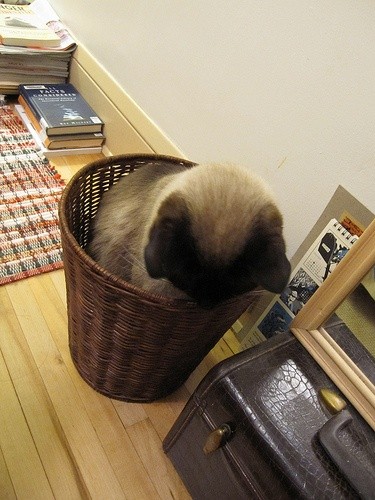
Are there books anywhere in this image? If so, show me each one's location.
[14,83,106,158]
[0,0,76,94]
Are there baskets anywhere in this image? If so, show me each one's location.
[58,153,265,404]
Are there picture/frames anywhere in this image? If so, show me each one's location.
[289,218,375,429]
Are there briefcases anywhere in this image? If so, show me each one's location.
[323,312,375,386]
[161,330,375,500]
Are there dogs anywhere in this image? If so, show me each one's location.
[89,159,292,314]
